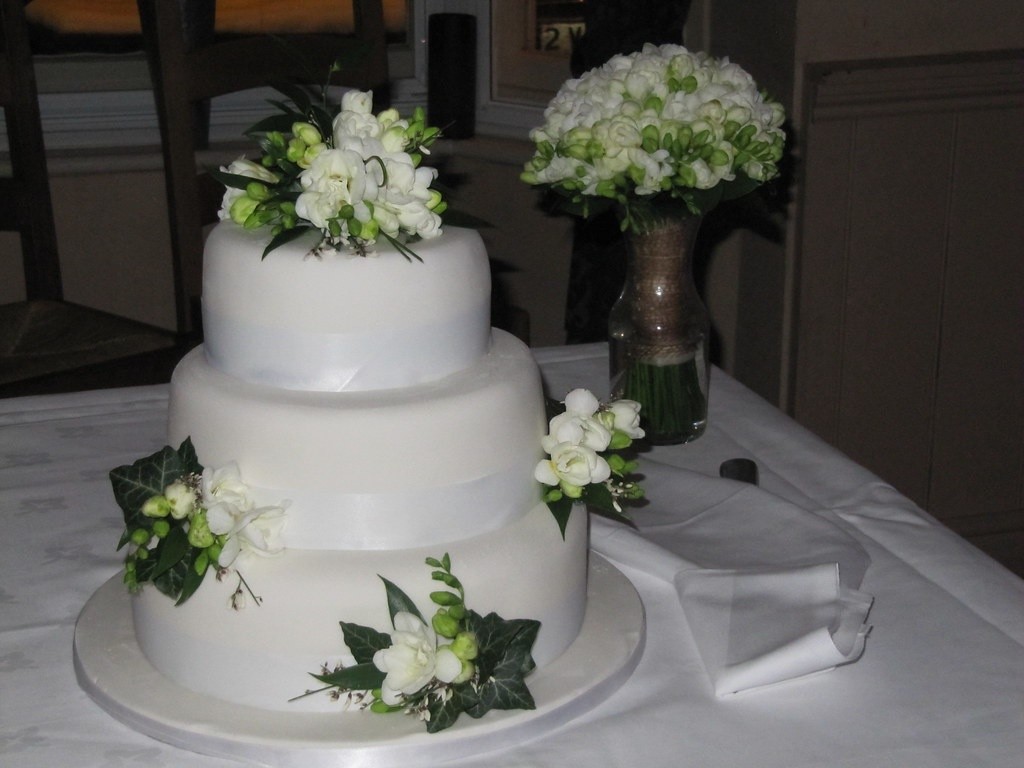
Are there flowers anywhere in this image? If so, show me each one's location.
[198,31,455,262]
[108,434,291,612]
[519,45,789,436]
[536,386,652,544]
[285,555,541,735]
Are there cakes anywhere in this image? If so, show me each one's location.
[108,78,651,733]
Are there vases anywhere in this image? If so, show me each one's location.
[605,199,712,445]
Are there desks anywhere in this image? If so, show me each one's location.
[0,343,1024,768]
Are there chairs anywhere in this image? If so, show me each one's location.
[135,0,531,349]
[0,0,197,399]
